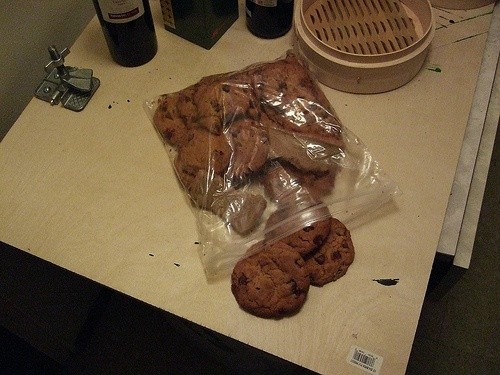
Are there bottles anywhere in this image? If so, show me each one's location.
[93,0,159,68]
[245,0,295,40]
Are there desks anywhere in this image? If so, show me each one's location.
[0,2,494,375]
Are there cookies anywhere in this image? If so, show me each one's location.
[153,49,355,319]
